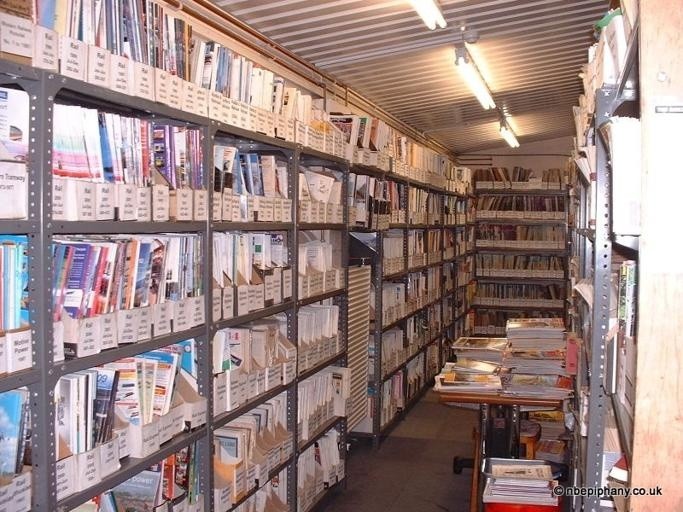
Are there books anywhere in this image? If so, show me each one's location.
[0,0,636,511]
